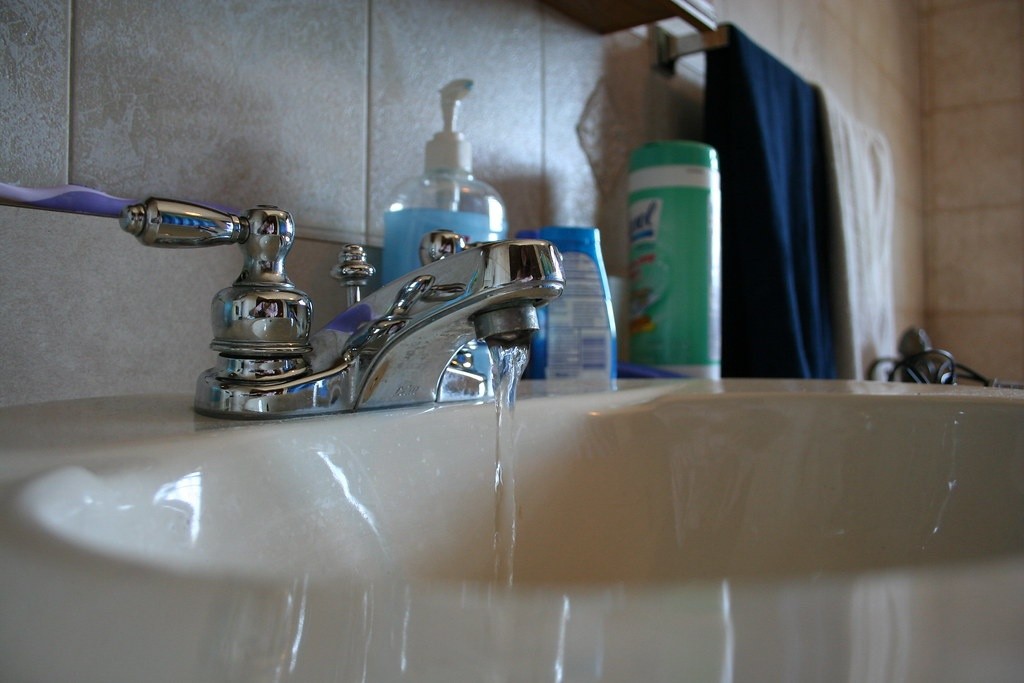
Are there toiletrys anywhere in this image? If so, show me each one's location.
[378,77,510,398]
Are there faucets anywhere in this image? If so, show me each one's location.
[118,195,568,422]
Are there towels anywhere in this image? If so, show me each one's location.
[700,21,900,382]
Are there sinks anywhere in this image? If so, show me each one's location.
[14,385,1024,591]
